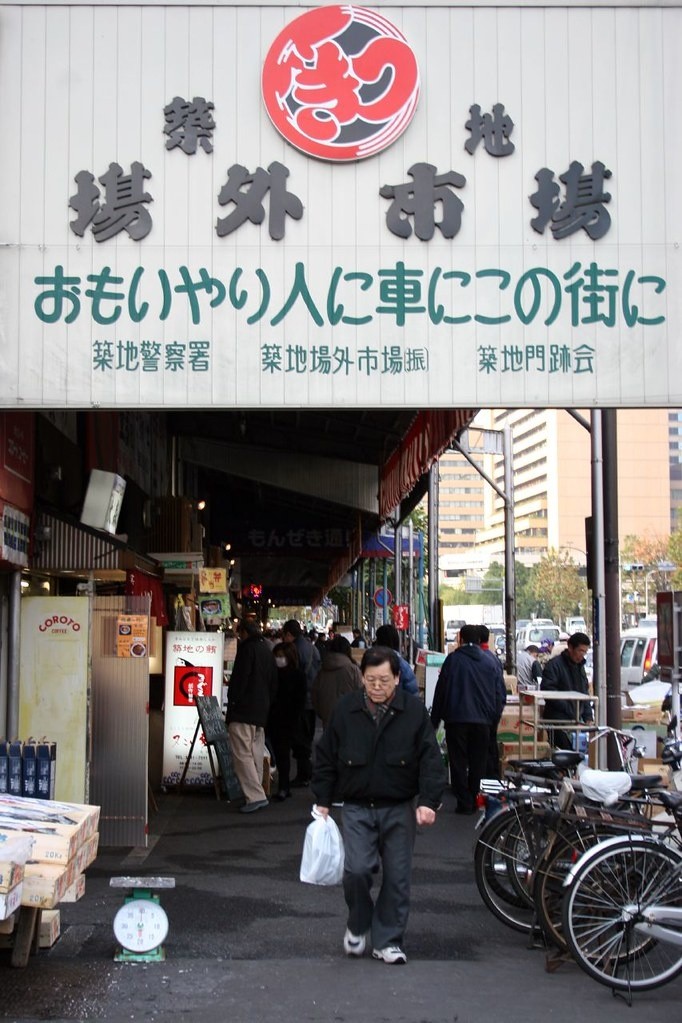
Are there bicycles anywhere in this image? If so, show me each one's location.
[473,725,682,1007]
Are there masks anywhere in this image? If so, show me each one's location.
[275,657,287,668]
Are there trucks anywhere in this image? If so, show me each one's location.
[565,617,586,637]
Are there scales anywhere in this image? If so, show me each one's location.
[109,876,175,961]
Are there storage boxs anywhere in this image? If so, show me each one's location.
[0,792,102,950]
[81,468,127,535]
[415,648,442,666]
[416,666,670,814]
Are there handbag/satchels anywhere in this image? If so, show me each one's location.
[299,806,346,885]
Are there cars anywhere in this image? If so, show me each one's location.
[486,618,593,683]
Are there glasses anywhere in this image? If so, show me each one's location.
[573,647,588,657]
[362,675,395,692]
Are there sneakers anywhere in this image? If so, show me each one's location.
[241,797,269,813]
[343,922,365,957]
[373,942,406,964]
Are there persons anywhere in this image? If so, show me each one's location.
[517,645,543,686]
[265,619,320,799]
[427,626,505,813]
[312,646,445,965]
[221,617,273,811]
[541,632,594,748]
[304,627,368,654]
[538,632,568,664]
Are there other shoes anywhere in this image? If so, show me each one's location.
[298,773,309,789]
[276,787,291,803]
[454,804,471,814]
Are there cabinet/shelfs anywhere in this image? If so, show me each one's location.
[517,690,600,771]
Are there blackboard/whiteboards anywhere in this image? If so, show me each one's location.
[195,696,245,800]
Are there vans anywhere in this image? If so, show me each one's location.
[444,618,467,642]
[620,628,658,693]
[638,614,657,629]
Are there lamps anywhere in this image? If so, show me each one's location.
[221,541,232,550]
[191,495,206,511]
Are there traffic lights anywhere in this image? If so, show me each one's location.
[630,564,644,572]
